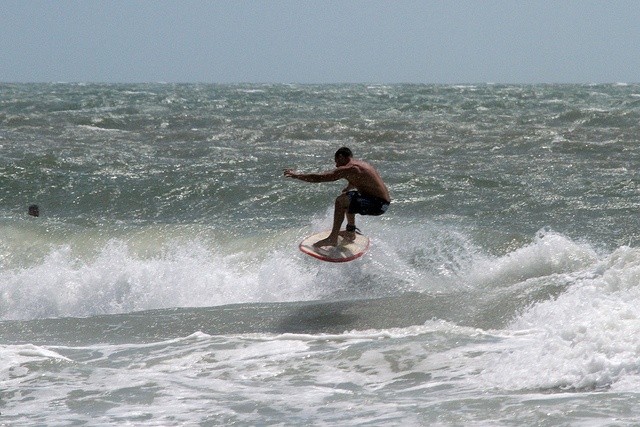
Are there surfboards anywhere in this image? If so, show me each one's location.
[299,229,370,262]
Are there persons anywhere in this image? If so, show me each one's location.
[282,147,390,248]
[28,205,39,218]
